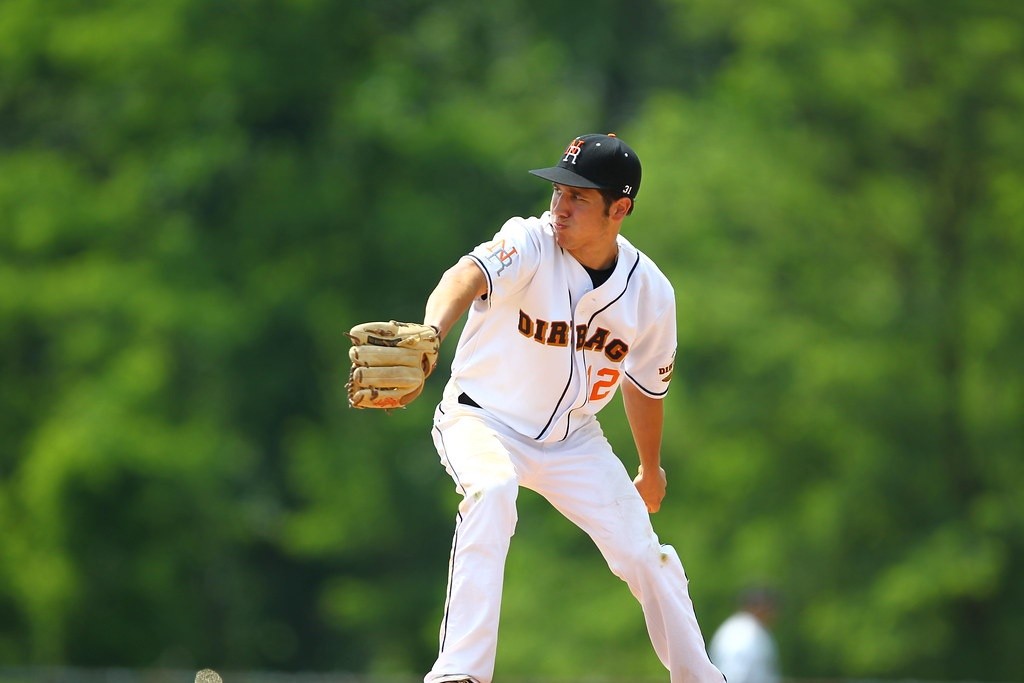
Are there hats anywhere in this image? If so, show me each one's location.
[529,133,642,198]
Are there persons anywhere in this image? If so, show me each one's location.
[345,127,729,683]
[710,580,786,683]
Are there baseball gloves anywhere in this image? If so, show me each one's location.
[347,318,441,411]
